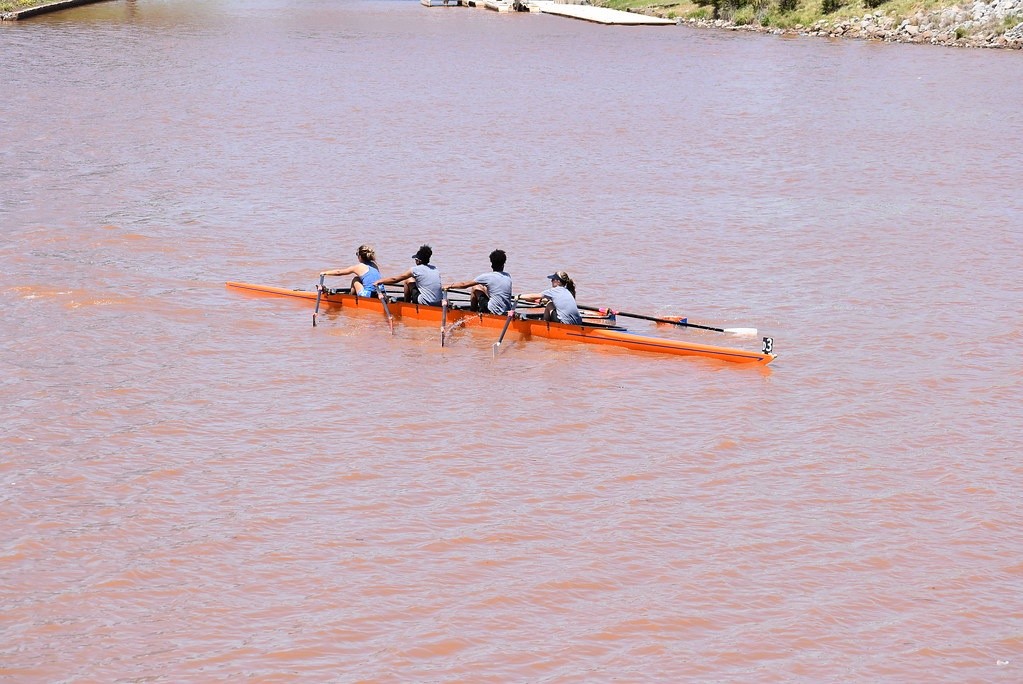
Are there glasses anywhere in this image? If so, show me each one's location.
[356,252,359,256]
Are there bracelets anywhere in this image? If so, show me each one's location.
[451,283,454,289]
[518,294,521,299]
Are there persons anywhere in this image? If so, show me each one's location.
[373,244,442,306]
[442,249,512,316]
[320,245,386,298]
[515,272,582,325]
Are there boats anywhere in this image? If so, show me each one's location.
[222,278,780,372]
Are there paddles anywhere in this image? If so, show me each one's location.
[312,276,324,327]
[492,299,518,358]
[440,289,447,348]
[384,283,536,305]
[374,286,393,336]
[511,296,758,337]
[336,287,404,293]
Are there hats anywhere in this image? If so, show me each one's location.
[412,251,429,261]
[547,272,566,285]
